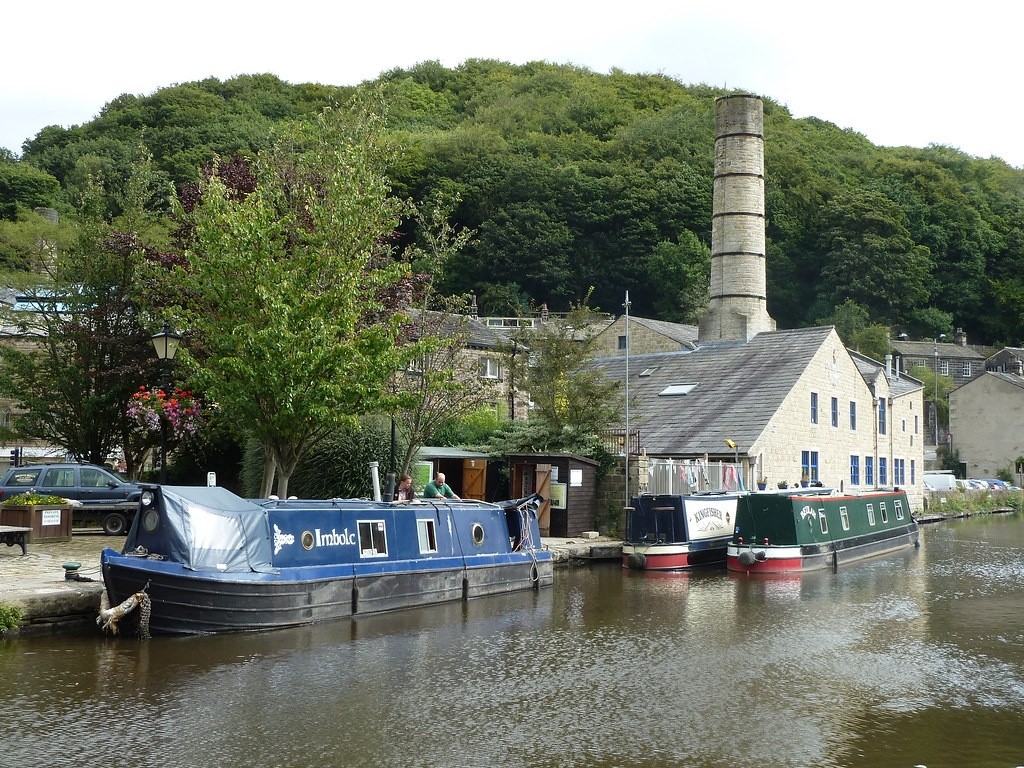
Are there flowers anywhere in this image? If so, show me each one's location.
[2,488,67,505]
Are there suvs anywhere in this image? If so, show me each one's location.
[0,460,142,504]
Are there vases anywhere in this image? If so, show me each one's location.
[0,504,73,543]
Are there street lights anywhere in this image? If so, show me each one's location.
[151,323,183,485]
[886,333,907,355]
[934,334,946,448]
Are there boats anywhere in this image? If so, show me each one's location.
[101,462,553,635]
[622,489,920,573]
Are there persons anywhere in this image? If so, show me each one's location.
[393,474,414,501]
[424,473,460,500]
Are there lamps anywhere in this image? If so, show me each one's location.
[140,490,155,507]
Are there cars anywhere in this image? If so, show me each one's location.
[955,479,1021,491]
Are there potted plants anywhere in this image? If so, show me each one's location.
[777,480,788,489]
[757,475,768,490]
[801,475,809,487]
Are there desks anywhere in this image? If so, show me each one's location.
[0,525,33,556]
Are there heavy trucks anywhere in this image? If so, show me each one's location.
[72,502,139,536]
